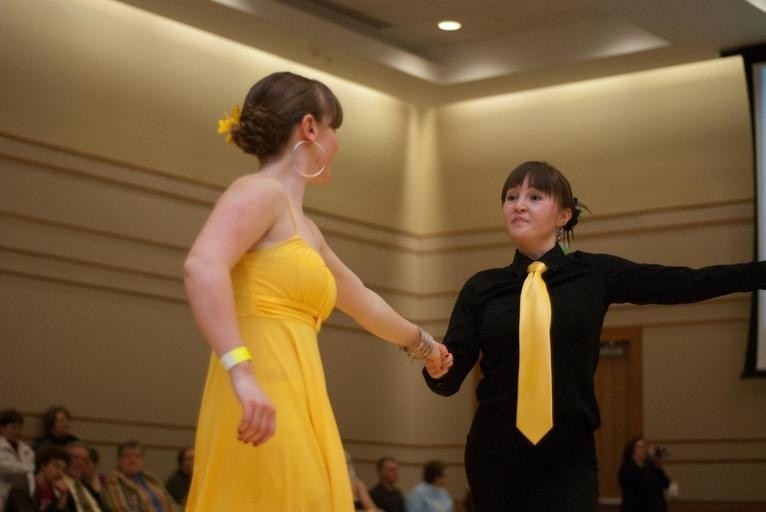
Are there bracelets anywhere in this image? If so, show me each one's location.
[217,346,250,370]
[398,327,436,363]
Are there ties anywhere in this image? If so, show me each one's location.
[515,260,555,447]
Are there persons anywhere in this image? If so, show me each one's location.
[358,455,407,511]
[404,458,457,511]
[180,71,451,512]
[0,401,194,512]
[618,436,672,510]
[420,160,765,511]
[345,451,385,512]
